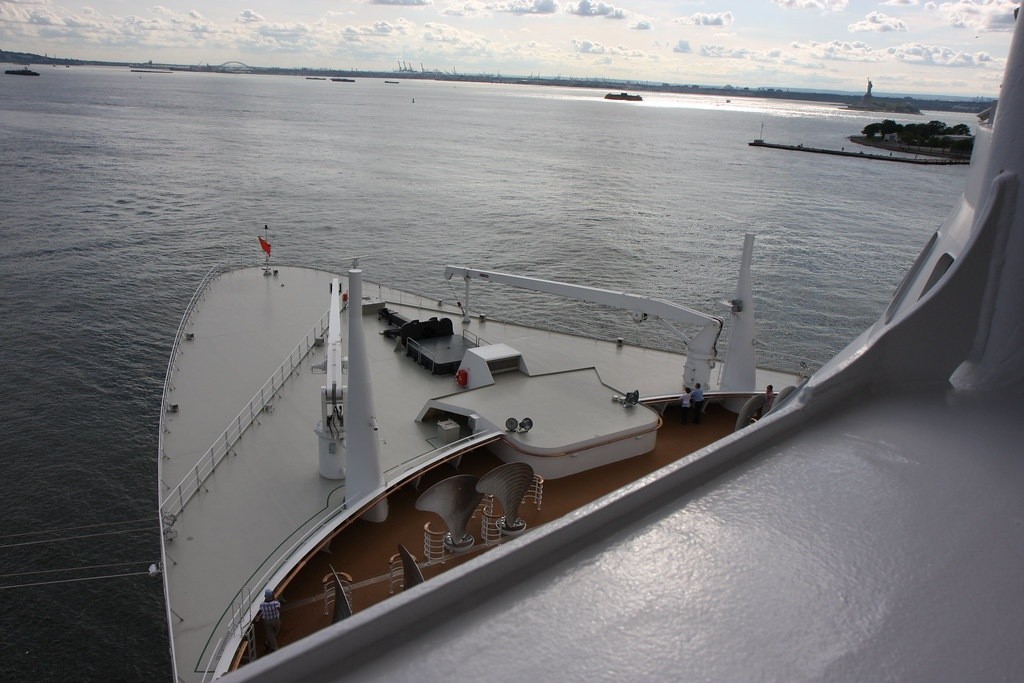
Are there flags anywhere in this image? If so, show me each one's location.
[258,236,270,257]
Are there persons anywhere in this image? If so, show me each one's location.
[765,385,774,406]
[691,383,704,424]
[678,388,691,424]
[259,589,280,651]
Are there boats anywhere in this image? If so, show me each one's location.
[604,92,642,102]
[4,69,41,76]
